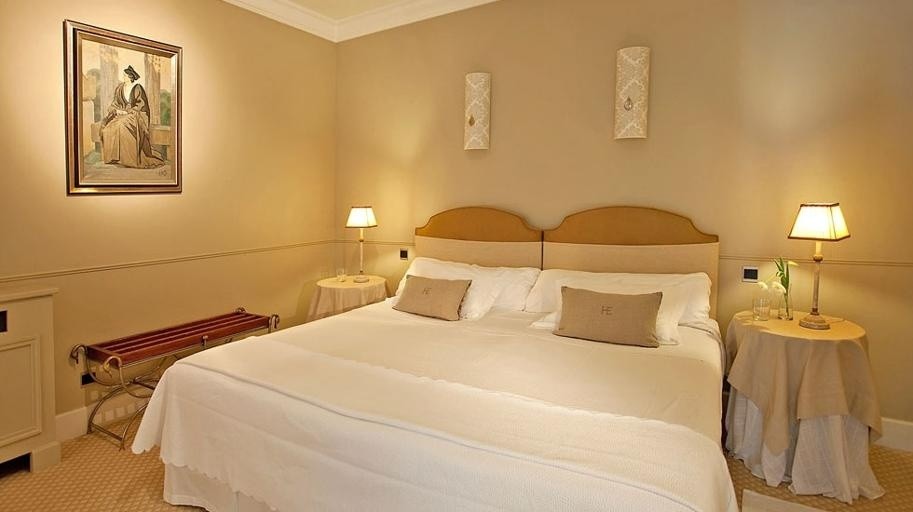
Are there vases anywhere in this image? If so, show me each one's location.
[777,294,793,321]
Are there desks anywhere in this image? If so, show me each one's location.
[732,309,867,484]
[313,274,387,317]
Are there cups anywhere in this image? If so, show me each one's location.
[751,299,772,322]
[336,268,346,282]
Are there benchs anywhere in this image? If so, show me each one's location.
[68,305,281,451]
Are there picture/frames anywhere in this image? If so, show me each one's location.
[62,19,182,196]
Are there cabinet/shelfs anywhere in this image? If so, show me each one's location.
[0,285,62,475]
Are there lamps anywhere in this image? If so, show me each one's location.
[612,46,650,141]
[464,72,489,151]
[787,202,850,332]
[344,204,378,283]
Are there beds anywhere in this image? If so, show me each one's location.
[162,206,726,512]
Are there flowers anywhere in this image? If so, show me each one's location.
[755,255,799,317]
